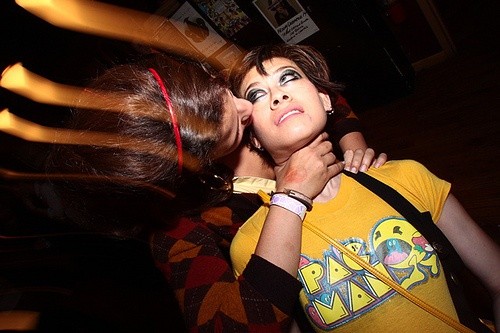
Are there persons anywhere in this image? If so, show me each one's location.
[47,52,387,333]
[229,42,500,333]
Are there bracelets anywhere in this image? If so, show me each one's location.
[269,189,313,211]
[269,194,307,222]
